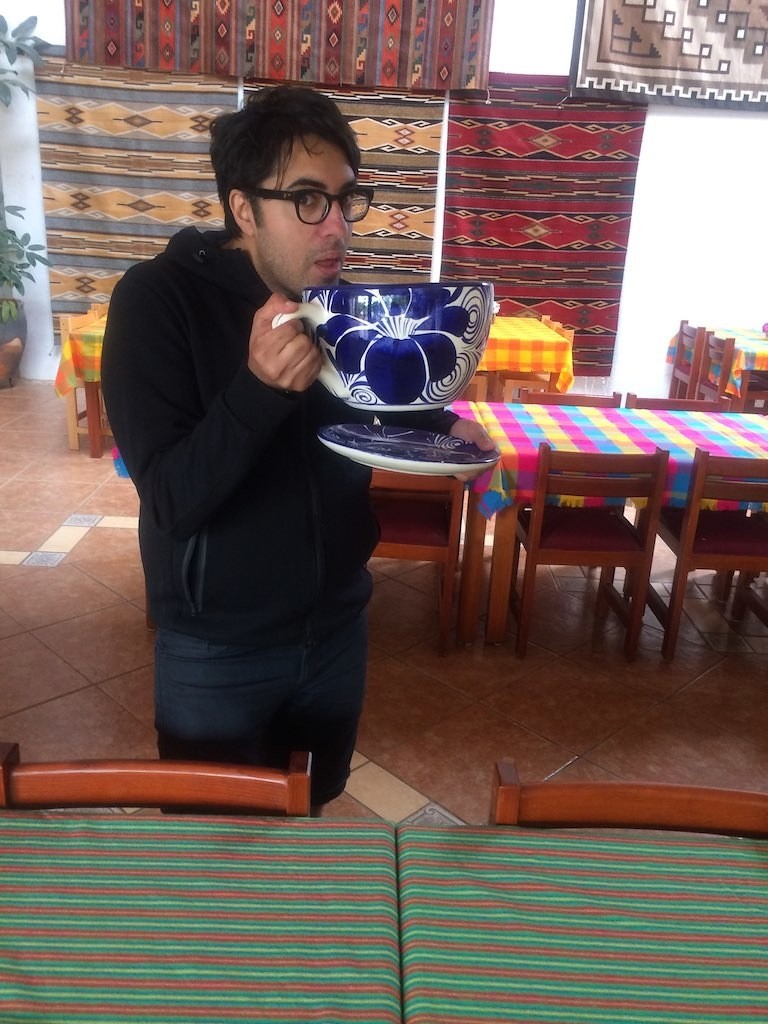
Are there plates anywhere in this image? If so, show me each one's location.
[317,421,501,476]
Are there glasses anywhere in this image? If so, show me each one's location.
[243,186,374,226]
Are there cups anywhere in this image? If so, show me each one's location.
[273,280,500,413]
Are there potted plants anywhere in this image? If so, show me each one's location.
[0,12,51,387]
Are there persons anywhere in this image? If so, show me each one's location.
[99,83,500,815]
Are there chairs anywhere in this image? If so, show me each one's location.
[0,738,313,817]
[487,761,768,841]
[368,466,463,646]
[475,316,768,670]
[60,301,114,450]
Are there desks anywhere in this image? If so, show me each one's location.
[477,402,768,646]
[474,317,566,401]
[68,315,111,458]
[403,825,768,1024]
[0,814,396,1024]
[706,328,768,411]
